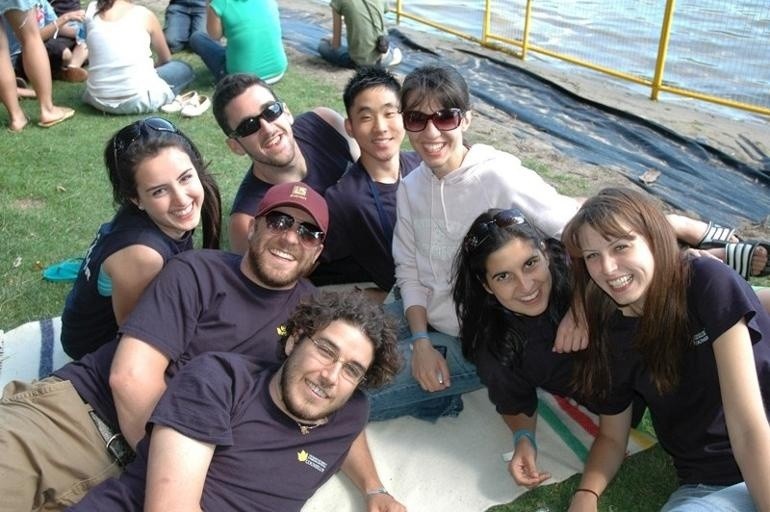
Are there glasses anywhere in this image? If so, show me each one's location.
[114,112,181,196]
[400,105,467,133]
[465,206,533,252]
[229,99,286,140]
[256,209,324,249]
[300,327,369,386]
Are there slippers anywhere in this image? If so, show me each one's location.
[6,113,31,134]
[42,256,86,283]
[35,104,76,132]
[700,237,769,282]
[673,211,743,254]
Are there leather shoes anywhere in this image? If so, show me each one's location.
[179,94,212,119]
[157,88,197,113]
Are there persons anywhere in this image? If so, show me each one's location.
[358,62,588,424]
[0,182,330,511]
[0,0,287,135]
[71,285,410,512]
[60,117,220,360]
[321,67,769,294]
[212,74,363,261]
[457,207,770,486]
[563,187,769,511]
[318,1,391,70]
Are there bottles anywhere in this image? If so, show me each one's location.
[75,21,87,45]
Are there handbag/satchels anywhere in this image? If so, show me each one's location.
[374,35,390,54]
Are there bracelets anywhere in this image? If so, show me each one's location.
[410,331,432,341]
[364,488,392,497]
[571,487,602,498]
[511,430,538,455]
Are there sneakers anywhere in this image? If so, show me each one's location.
[52,64,90,84]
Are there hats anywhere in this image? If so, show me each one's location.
[250,181,330,235]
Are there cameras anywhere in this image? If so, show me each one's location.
[377,35,389,53]
